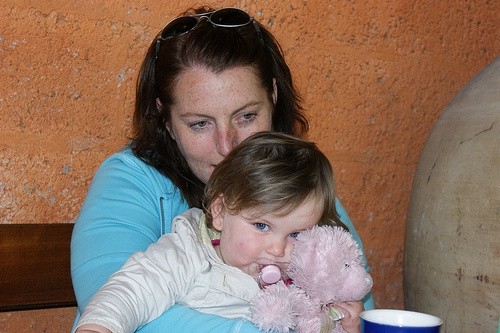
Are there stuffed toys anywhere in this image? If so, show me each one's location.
[250,225,373,332]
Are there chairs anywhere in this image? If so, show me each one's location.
[0,223,79,312]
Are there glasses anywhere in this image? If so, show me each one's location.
[155,6,267,80]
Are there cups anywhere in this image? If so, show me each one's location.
[359,309,443,333]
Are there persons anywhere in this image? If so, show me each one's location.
[70,5,376,332]
[74,132,364,332]
